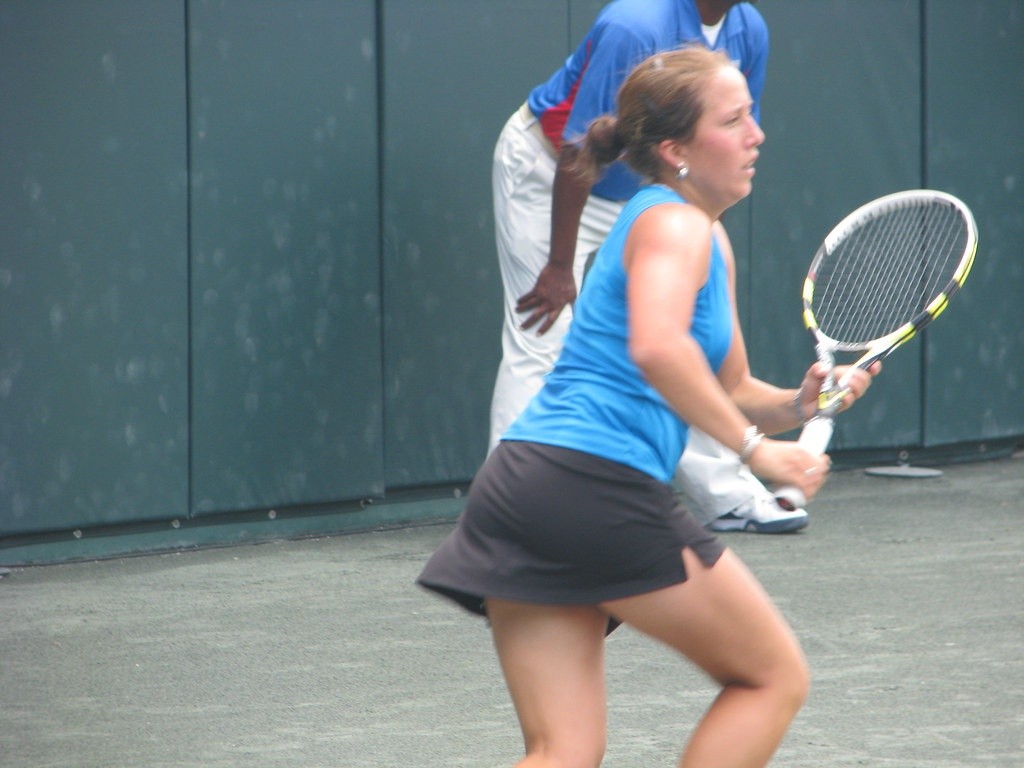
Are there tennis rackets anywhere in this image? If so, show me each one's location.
[774,188,980,513]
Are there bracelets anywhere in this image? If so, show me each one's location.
[794,390,808,423]
[738,426,766,463]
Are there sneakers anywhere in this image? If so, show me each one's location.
[711,493,809,534]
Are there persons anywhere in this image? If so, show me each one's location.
[490,0,810,533]
[415,46,881,768]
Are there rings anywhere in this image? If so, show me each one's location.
[805,467,816,475]
